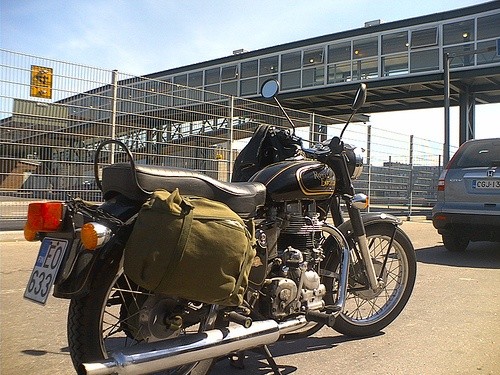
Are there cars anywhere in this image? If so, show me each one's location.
[431,137,500,252]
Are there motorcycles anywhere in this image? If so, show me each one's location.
[22,78,418,375]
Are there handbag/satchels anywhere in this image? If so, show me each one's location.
[124,189,257,306]
[231,123,292,181]
